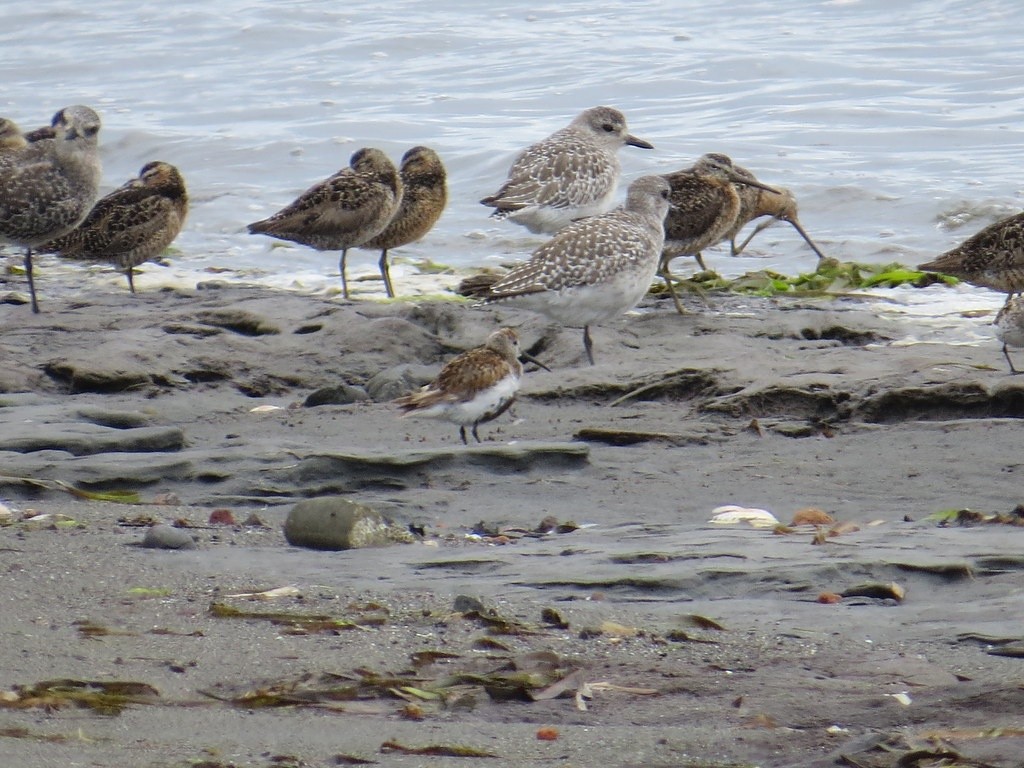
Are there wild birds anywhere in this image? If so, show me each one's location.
[246,148,401,300]
[694,163,827,276]
[468,175,682,367]
[569,152,782,316]
[391,327,552,446]
[915,211,1024,375]
[356,146,448,301]
[0,105,100,314]
[32,160,188,293]
[478,106,654,238]
